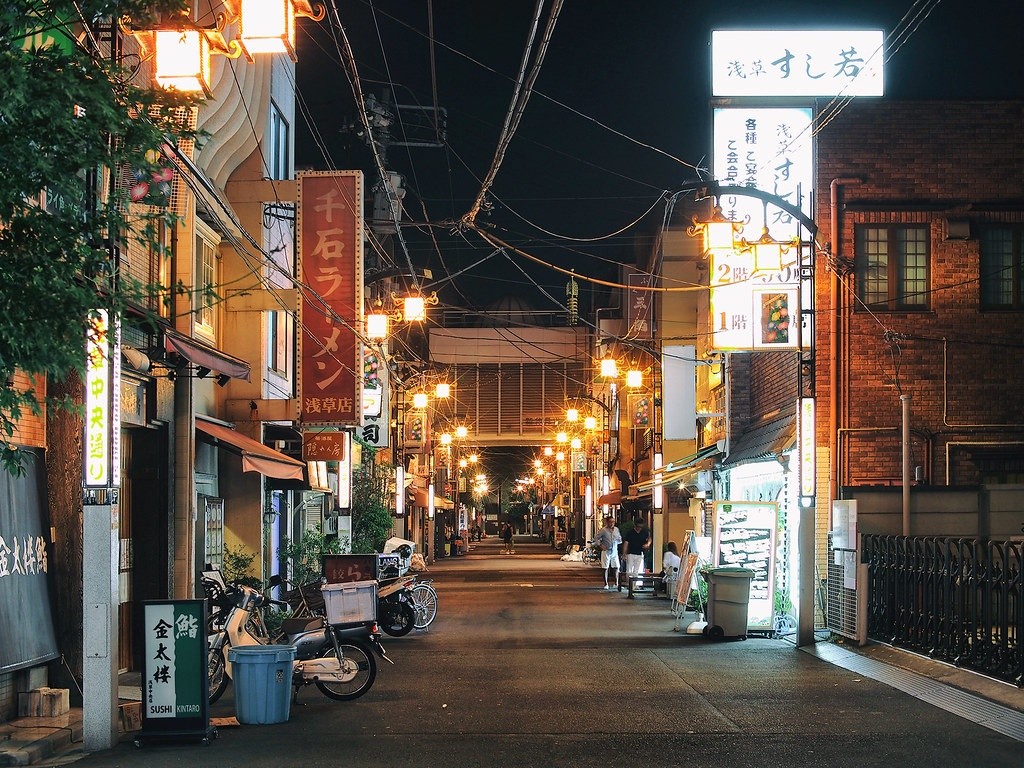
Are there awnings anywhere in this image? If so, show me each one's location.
[195,416,307,480]
[542,502,558,515]
[552,493,560,507]
[599,492,622,504]
[413,486,444,507]
[628,461,709,492]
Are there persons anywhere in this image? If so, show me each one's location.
[505,521,514,555]
[621,518,652,589]
[594,516,622,589]
[654,542,681,597]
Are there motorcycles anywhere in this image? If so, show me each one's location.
[319,574,420,637]
[200,570,395,708]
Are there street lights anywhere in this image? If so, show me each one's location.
[337,267,440,555]
[428,412,469,567]
[679,177,817,647]
[394,362,450,537]
[567,393,612,522]
[597,333,667,589]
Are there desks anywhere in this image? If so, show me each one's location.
[618,571,662,593]
[628,575,663,599]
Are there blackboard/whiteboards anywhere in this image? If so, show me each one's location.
[377,557,399,602]
[674,529,692,594]
[678,553,698,605]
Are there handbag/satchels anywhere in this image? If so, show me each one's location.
[499,525,507,538]
[511,538,514,547]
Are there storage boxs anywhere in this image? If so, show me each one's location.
[18,692,31,716]
[31,687,51,717]
[320,580,379,624]
[119,701,143,731]
[382,536,416,568]
[378,553,400,580]
[43,689,69,716]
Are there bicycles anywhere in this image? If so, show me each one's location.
[398,577,438,633]
[243,567,329,645]
[582,541,601,563]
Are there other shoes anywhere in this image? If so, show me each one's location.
[613,584,624,589]
[604,585,609,589]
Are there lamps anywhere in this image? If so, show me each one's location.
[686,191,743,259]
[733,200,801,281]
[121,344,149,371]
[390,277,439,323]
[118,0,242,100]
[148,362,211,379]
[601,345,616,377]
[157,353,190,371]
[222,0,326,64]
[627,349,642,388]
[135,343,166,361]
[169,371,231,387]
[364,281,403,337]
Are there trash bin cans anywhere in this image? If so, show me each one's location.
[227,644,298,725]
[698,567,755,641]
